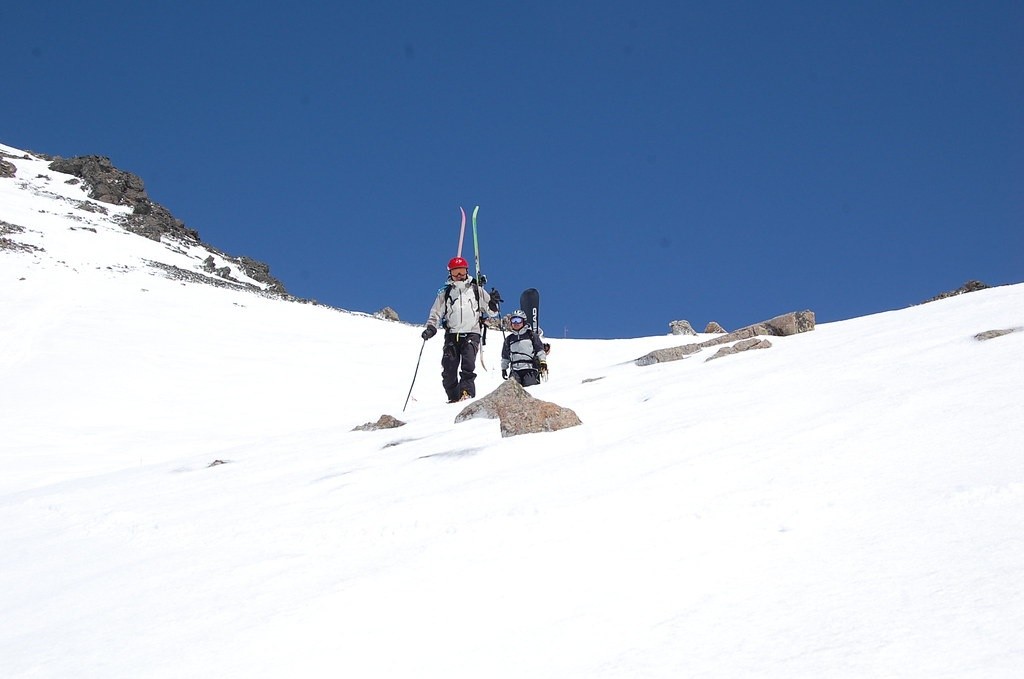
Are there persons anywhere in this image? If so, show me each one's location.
[421,256,500,404]
[501,310,550,387]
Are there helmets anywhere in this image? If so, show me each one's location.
[510,310,527,322]
[447,257,468,270]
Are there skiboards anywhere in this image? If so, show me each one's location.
[456,205,487,288]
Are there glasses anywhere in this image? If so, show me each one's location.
[510,317,523,324]
[449,268,468,277]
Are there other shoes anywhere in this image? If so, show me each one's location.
[460,391,472,400]
[447,399,459,404]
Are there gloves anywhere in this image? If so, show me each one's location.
[501,370,508,380]
[421,325,437,340]
[488,291,501,311]
[540,361,547,372]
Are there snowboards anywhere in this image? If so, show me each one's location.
[520,287,540,333]
